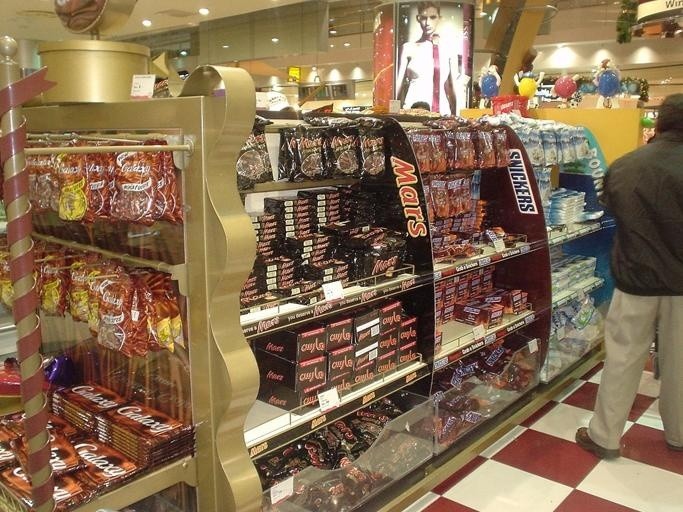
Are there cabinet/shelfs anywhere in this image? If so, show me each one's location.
[232,113,615,512]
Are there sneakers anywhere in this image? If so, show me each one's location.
[576,427,620,460]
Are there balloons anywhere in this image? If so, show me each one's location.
[518,78,538,100]
[554,76,578,98]
[597,71,619,98]
[481,75,499,100]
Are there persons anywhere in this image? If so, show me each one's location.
[576,90,683,460]
[396,1,464,117]
